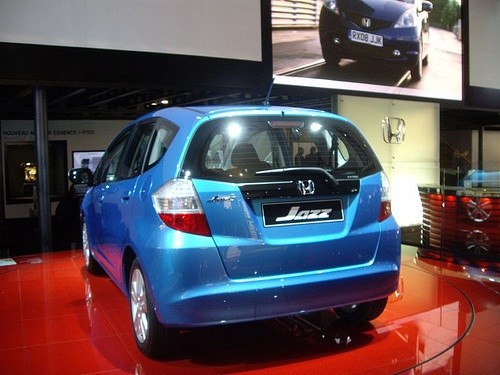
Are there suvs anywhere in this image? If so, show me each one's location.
[320,0,433,79]
[69,106,401,356]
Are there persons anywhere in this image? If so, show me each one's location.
[294,147,304,166]
[306,147,318,167]
